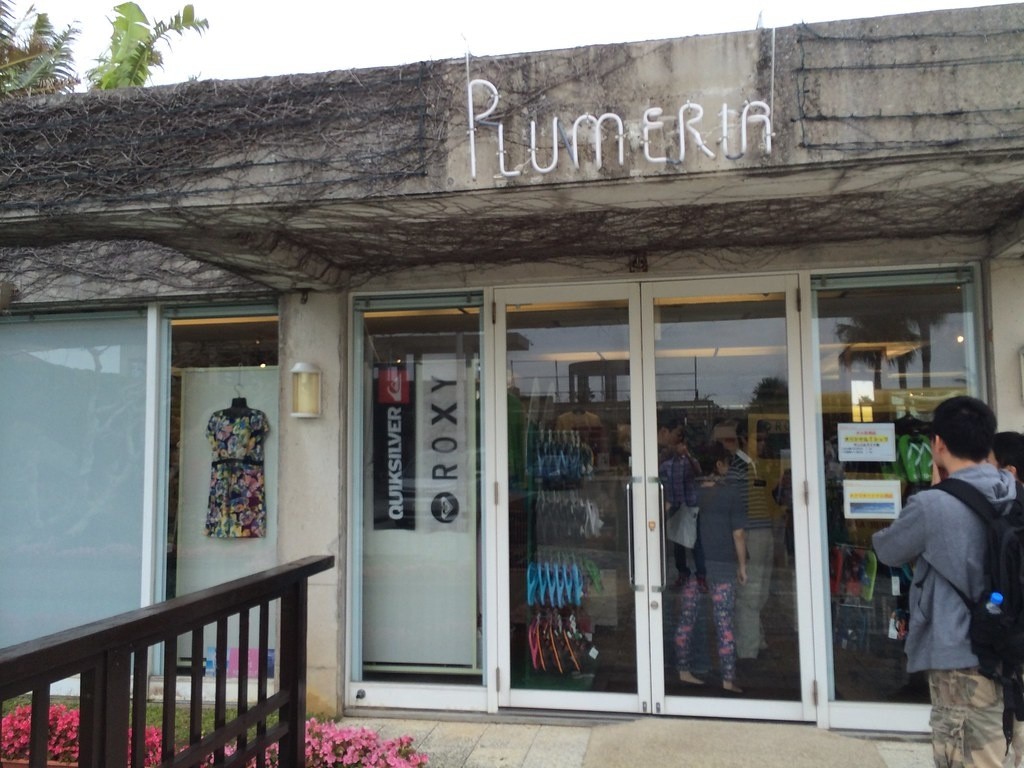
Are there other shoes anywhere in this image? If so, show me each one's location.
[723,688,746,697]
[675,577,690,586]
[696,578,708,594]
[681,679,712,688]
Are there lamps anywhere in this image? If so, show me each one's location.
[290,361,322,418]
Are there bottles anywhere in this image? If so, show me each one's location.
[986,592,1004,613]
[888,610,899,639]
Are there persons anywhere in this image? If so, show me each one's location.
[614,408,686,665]
[671,444,753,697]
[554,387,601,443]
[660,437,711,595]
[783,441,851,560]
[204,397,271,539]
[871,395,1017,768]
[985,430,1024,768]
[724,418,778,680]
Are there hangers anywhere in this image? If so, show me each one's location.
[571,403,585,413]
[229,382,247,409]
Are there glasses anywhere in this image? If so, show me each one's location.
[757,435,771,443]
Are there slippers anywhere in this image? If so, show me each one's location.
[824,433,934,659]
[527,437,605,673]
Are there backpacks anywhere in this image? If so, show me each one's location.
[925,478,1024,672]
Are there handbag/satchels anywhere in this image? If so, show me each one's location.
[664,501,700,549]
